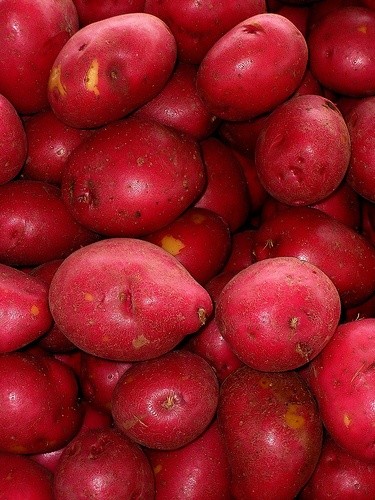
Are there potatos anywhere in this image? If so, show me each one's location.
[0,0,375,500]
